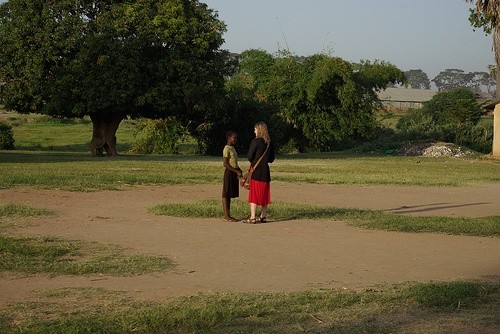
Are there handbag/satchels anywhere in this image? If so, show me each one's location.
[240,165,253,190]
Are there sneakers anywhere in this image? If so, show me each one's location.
[223,216,238,222]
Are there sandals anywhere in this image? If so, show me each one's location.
[243,217,256,224]
[256,217,267,223]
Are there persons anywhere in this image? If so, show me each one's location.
[242,122,275,224]
[222,130,243,221]
